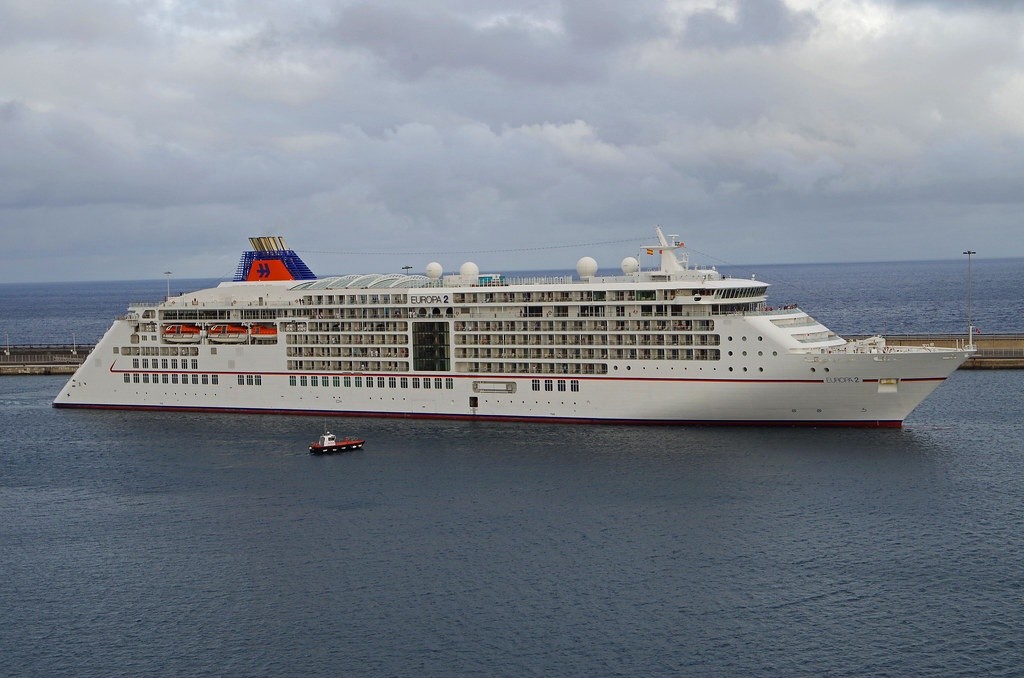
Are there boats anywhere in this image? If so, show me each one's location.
[307,416,366,455]
[161,324,201,344]
[207,324,248,342]
[251,325,277,341]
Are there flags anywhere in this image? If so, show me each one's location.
[646,248,654,256]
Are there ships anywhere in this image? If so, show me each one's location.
[49,223,984,429]
